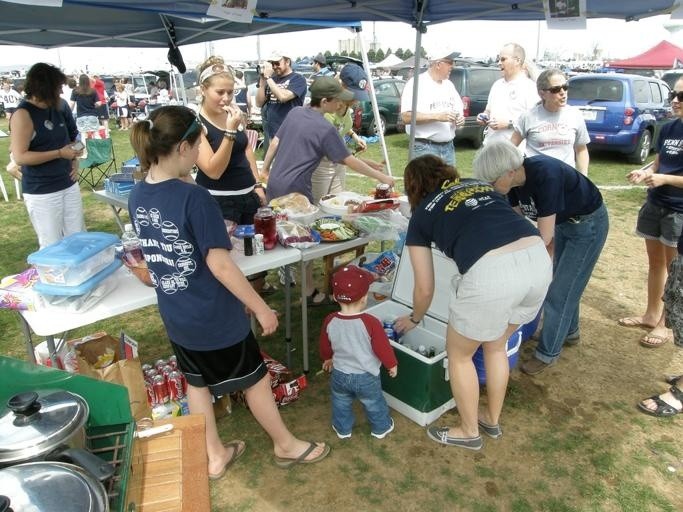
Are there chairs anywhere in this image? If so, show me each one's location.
[78,138,118,192]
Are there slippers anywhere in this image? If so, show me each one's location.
[640,331,673,348]
[209,440,247,479]
[619,316,656,328]
[275,440,330,467]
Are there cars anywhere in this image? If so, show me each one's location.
[348,79,407,138]
[562,65,624,79]
[659,69,683,92]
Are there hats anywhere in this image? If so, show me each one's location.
[309,77,354,101]
[314,55,326,65]
[331,265,373,303]
[266,48,292,62]
[340,65,370,101]
[428,47,461,61]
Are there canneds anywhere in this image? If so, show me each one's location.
[141,354,186,407]
[383,319,395,329]
[384,328,394,341]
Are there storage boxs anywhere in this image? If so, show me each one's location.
[31,257,123,314]
[521,300,543,342]
[474,331,523,385]
[72,336,151,423]
[359,240,462,428]
[26,231,119,287]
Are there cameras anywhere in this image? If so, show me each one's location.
[257,64,272,74]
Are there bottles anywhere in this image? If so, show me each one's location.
[224,183,412,259]
[382,320,435,358]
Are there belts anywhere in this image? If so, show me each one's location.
[415,139,453,145]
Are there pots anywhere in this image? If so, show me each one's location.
[0,388,113,483]
[0,458,109,512]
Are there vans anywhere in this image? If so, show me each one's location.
[565,71,679,164]
[394,59,504,150]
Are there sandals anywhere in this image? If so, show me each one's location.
[300,290,334,306]
[638,375,682,417]
[256,282,278,294]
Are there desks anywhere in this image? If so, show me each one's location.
[96,189,130,237]
[294,236,386,375]
[1,241,303,376]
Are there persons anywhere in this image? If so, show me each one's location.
[0,42,399,307]
[401,47,466,168]
[129,106,331,480]
[477,44,540,158]
[472,140,609,375]
[320,265,399,439]
[508,68,590,180]
[638,227,683,419]
[618,75,683,348]
[392,154,553,451]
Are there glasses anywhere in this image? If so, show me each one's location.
[177,115,201,150]
[543,80,569,94]
[212,65,235,73]
[668,91,683,102]
[497,58,506,62]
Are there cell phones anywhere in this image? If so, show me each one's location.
[72,140,84,151]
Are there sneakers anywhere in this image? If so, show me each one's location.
[530,326,580,345]
[521,357,549,375]
[478,421,503,439]
[278,266,296,286]
[427,426,482,450]
[371,417,395,439]
[331,425,351,439]
